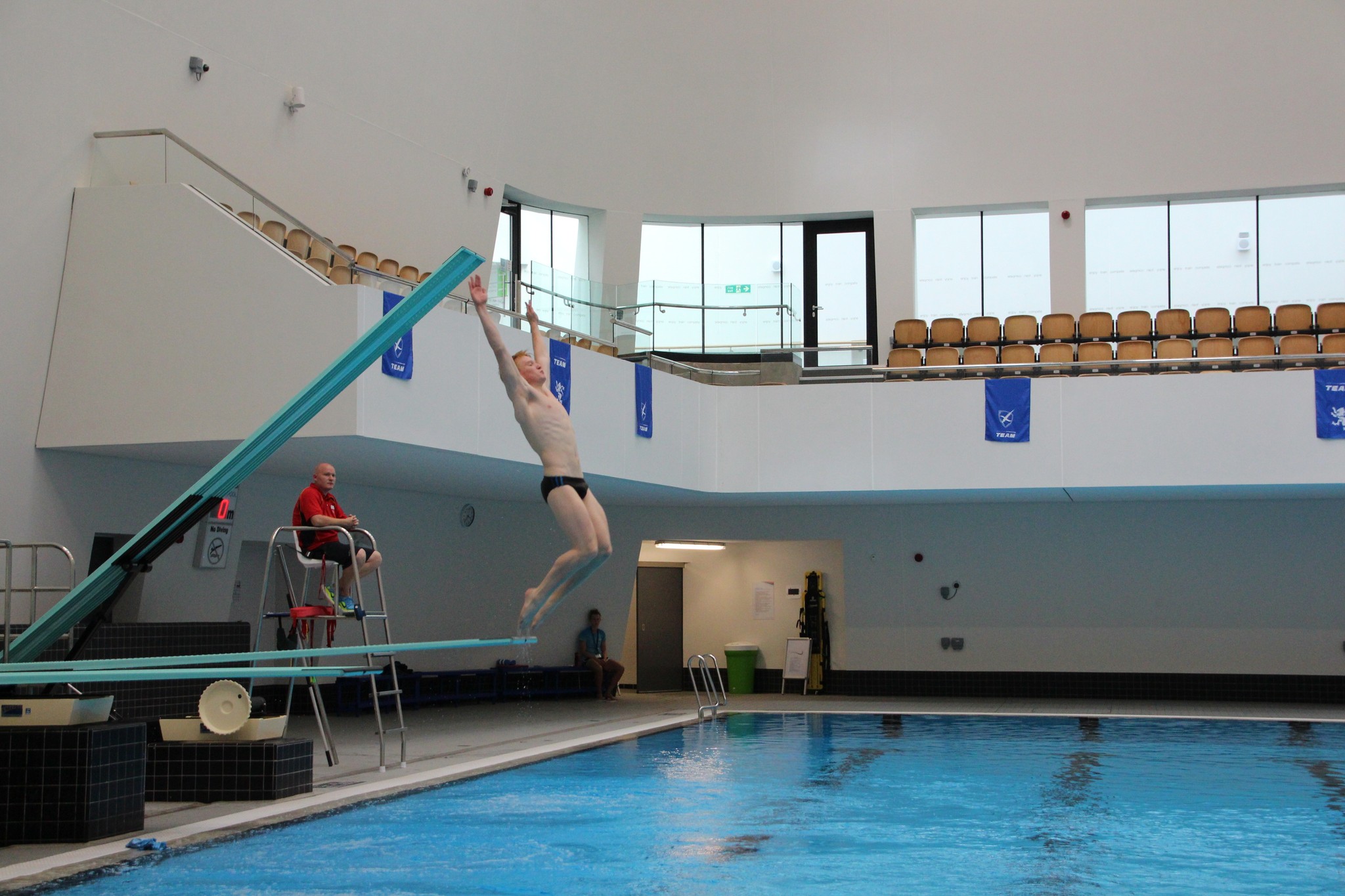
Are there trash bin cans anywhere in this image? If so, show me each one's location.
[723,641,760,695]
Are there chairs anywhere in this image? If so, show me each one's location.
[291,529,346,618]
[883,302,1345,368]
[541,329,619,359]
[224,204,437,291]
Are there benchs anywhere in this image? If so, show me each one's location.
[331,665,617,705]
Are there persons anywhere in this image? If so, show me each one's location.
[292,462,383,615]
[578,608,624,701]
[468,274,613,637]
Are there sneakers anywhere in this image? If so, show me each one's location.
[337,596,354,613]
[321,583,335,605]
